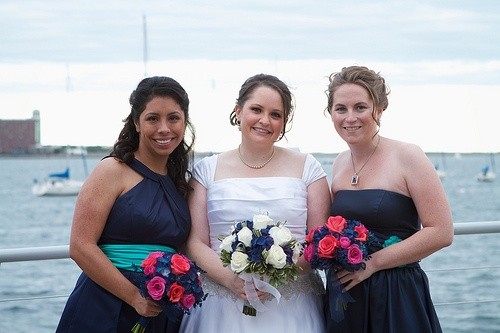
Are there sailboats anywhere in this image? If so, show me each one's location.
[478,152,493,180]
[31,148,88,198]
[427,129,451,178]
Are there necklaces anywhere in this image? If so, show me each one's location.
[238,144,276,169]
[350,135,381,185]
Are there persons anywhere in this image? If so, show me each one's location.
[56,74,196,333]
[323,65,456,333]
[178,73,333,333]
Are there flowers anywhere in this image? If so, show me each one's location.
[130,250,204,333]
[299,216,371,312]
[217,213,304,317]
